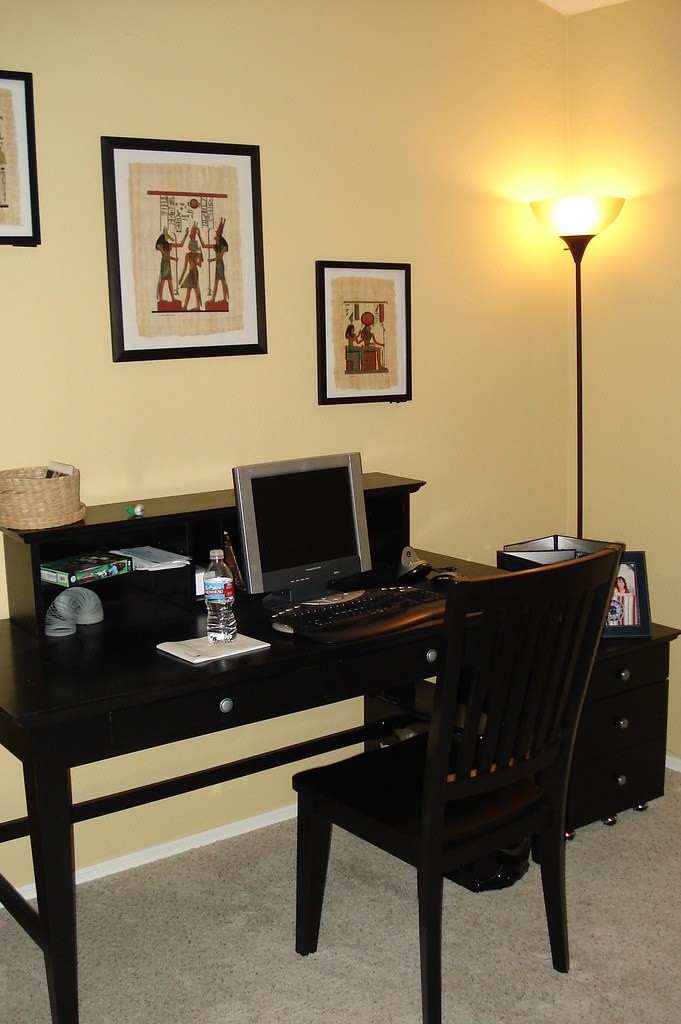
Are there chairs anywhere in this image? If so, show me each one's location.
[290,541,625,1024]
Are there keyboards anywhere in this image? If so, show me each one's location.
[266,586,448,646]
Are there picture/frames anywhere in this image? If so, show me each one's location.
[601,551,654,639]
[0,70,42,249]
[98,134,268,363]
[314,260,412,406]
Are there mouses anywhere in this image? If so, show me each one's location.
[431,571,468,588]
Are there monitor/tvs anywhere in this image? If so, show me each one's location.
[234,450,374,612]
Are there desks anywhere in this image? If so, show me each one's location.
[0,472,509,1024]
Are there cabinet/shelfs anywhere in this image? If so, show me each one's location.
[566,622,681,838]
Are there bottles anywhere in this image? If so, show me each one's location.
[204,550,237,644]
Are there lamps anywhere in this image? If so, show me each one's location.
[529,195,626,538]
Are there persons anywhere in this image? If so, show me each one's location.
[614,577,630,604]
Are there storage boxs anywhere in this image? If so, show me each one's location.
[40,551,134,587]
